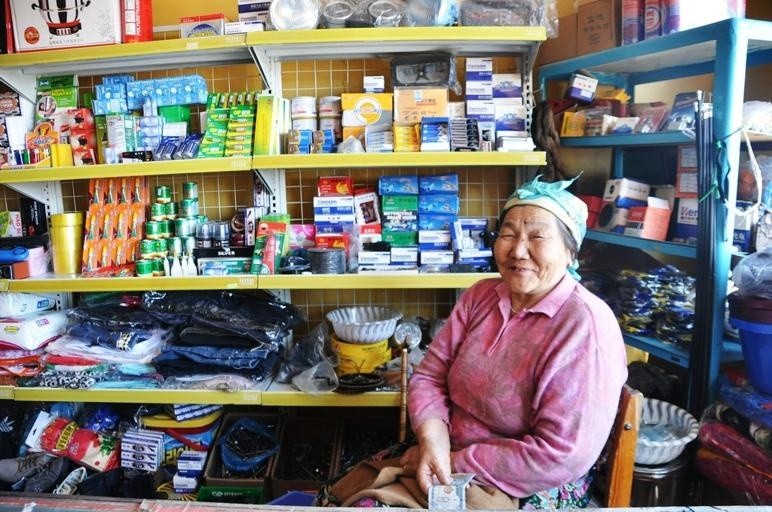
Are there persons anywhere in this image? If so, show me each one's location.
[38,101,56,118]
[310,173,629,510]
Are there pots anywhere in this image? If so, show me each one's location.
[32,1,89,35]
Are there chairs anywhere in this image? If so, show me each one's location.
[576,383,645,506]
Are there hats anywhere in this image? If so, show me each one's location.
[498,168,590,252]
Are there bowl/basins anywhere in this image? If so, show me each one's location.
[727,291,771,398]
[634,397,701,466]
[325,304,404,343]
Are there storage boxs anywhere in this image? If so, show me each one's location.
[572,0,623,60]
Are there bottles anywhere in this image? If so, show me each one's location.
[197,218,231,248]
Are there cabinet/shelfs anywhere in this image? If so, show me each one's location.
[0,0,547,511]
[534,16,772,508]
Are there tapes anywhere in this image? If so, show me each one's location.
[49,212,83,276]
